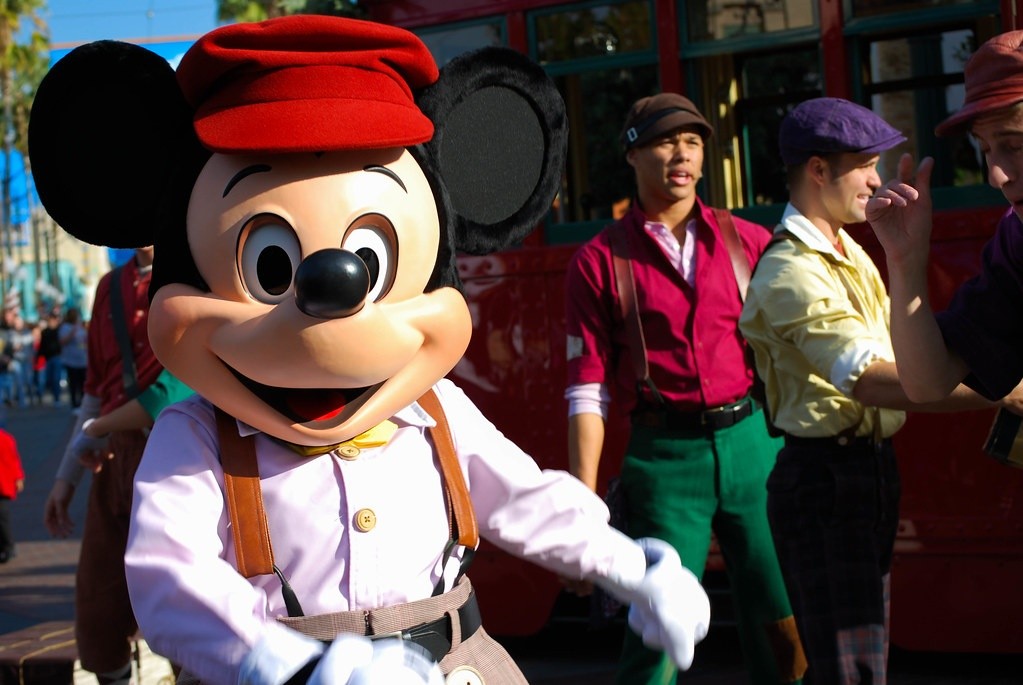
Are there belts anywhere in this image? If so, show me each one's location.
[640,401,752,431]
[784,433,892,452]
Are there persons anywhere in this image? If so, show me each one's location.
[44,243,198,685]
[27,16,710,685]
[738,97,1023,685]
[865,30,1023,404]
[557,93,809,685]
[0,428,27,563]
[0,295,89,415]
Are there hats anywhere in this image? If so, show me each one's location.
[779,96,908,162]
[620,93,715,156]
[935,30,1023,139]
